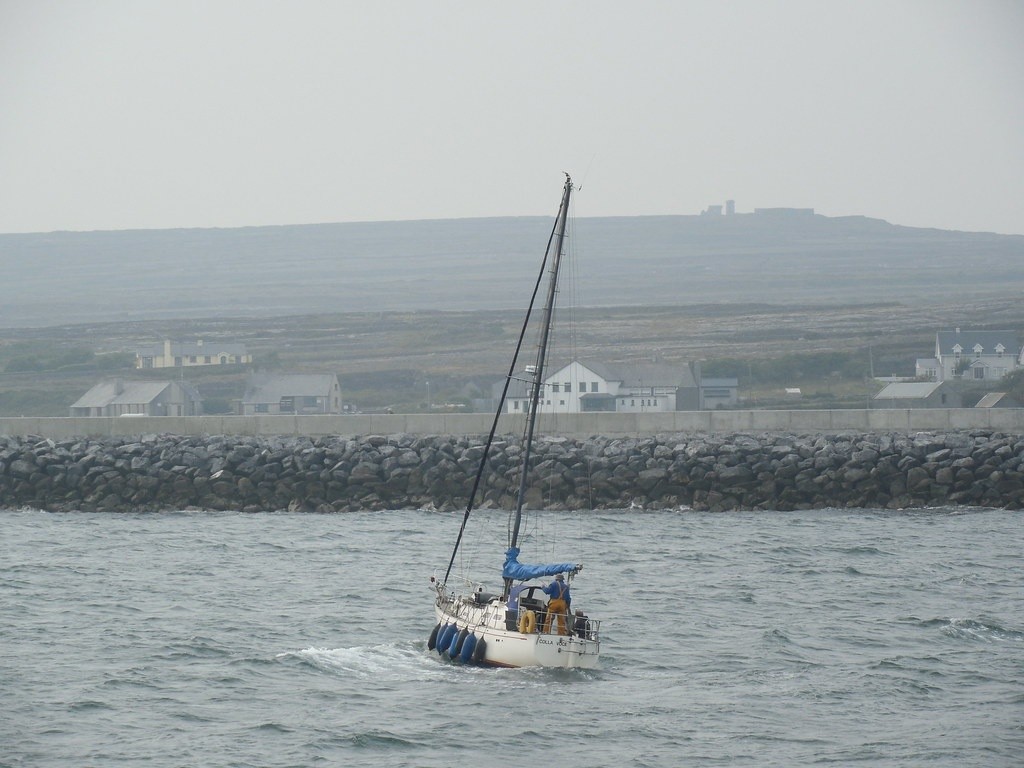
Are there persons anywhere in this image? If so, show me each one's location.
[571,608,591,639]
[541,574,571,636]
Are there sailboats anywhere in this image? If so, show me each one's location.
[424,168,604,675]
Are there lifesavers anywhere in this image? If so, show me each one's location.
[520,610,535,634]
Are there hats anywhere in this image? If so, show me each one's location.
[555,574,565,581]
